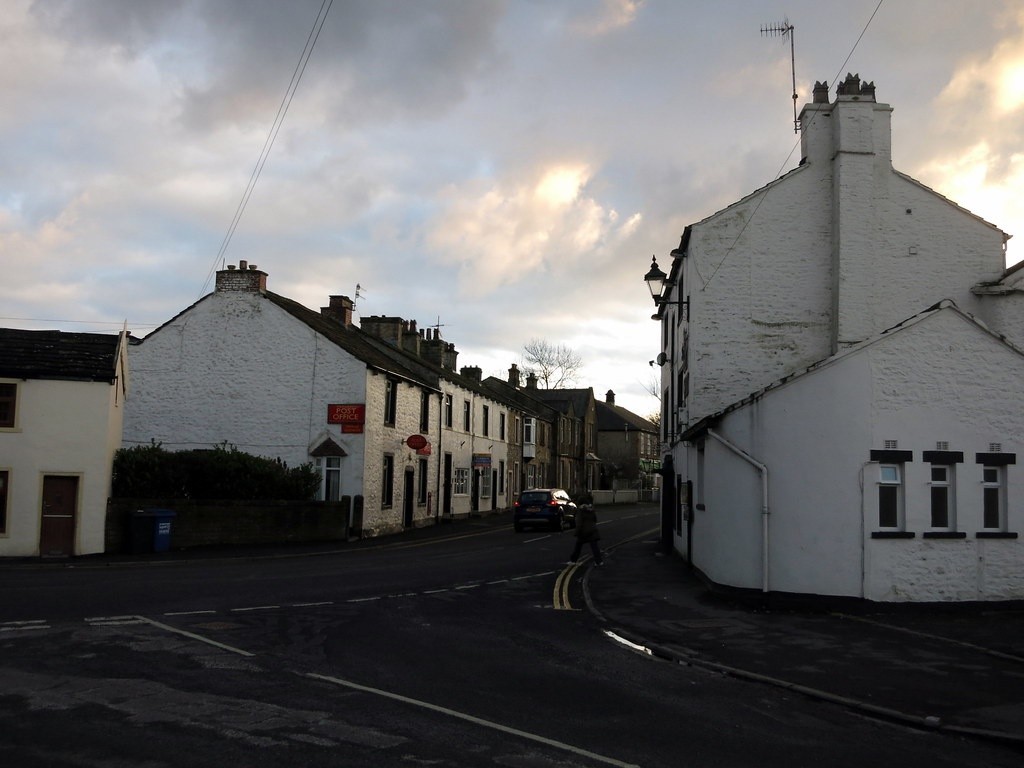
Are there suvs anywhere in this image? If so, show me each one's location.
[514,488,577,532]
